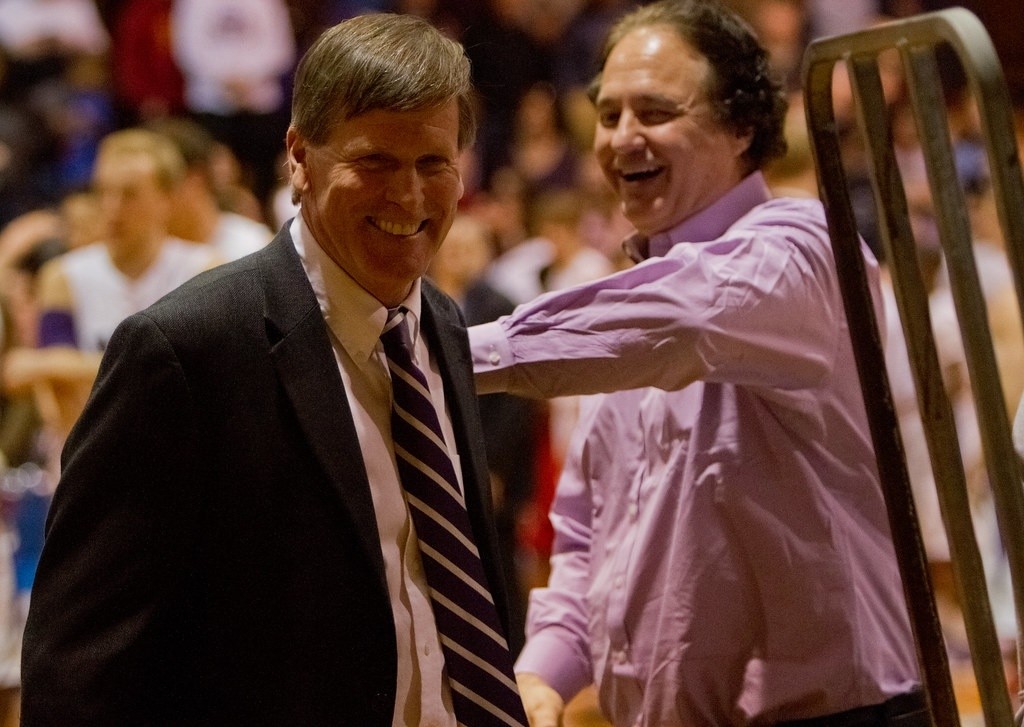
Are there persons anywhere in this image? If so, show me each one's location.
[0,0,1024,727]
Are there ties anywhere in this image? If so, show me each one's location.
[380,309,530,727]
